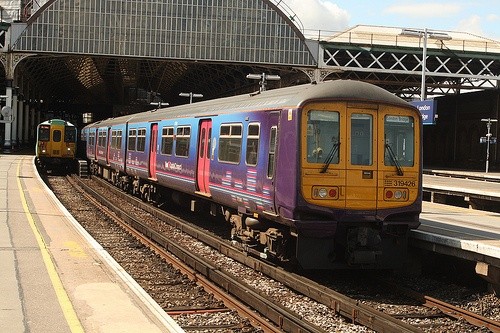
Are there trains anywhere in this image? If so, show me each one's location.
[35,118,88,175]
[82,73,424,277]
